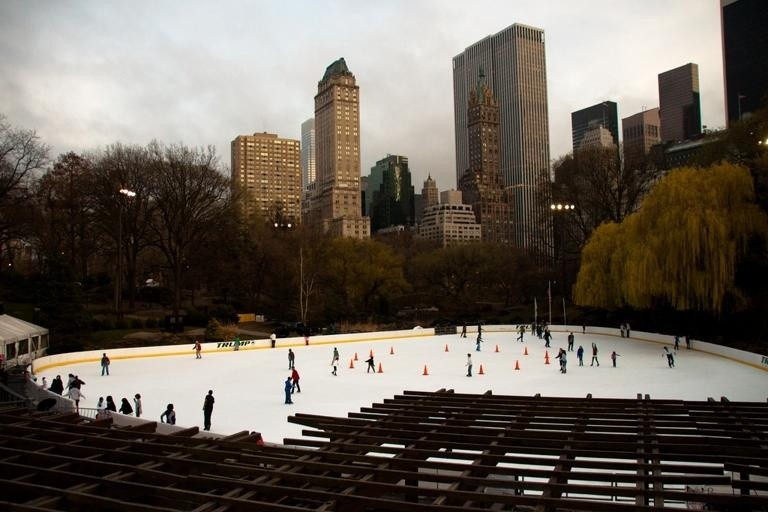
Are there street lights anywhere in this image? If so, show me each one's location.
[271,219,292,311]
[113,187,139,318]
[547,202,577,324]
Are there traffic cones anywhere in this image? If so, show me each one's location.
[343,343,552,377]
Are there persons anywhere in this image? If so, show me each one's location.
[203,391,215,431]
[365,357,376,373]
[686,335,691,349]
[661,346,677,368]
[288,349,295,370]
[332,358,339,376]
[290,367,301,394]
[270,332,276,348]
[460,320,630,377]
[331,348,339,365]
[674,336,682,350]
[234,337,240,351]
[285,377,294,404]
[192,341,201,359]
[41,353,176,425]
